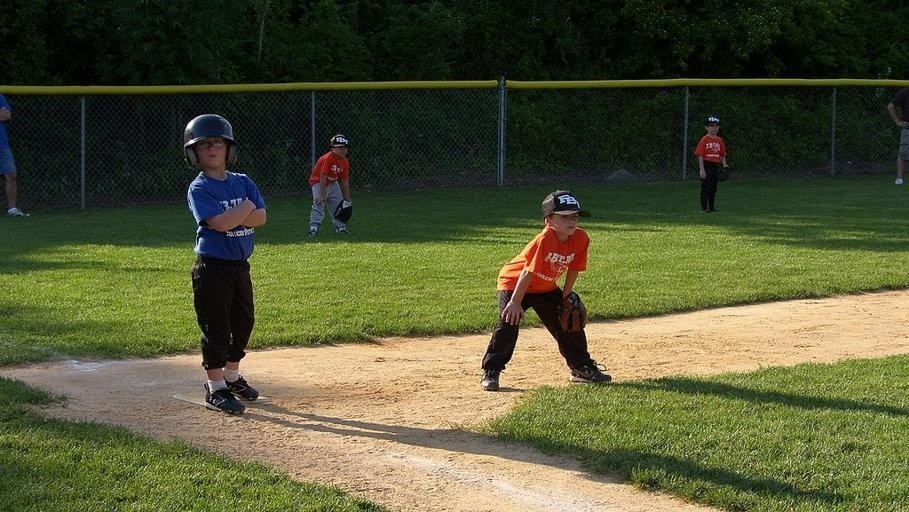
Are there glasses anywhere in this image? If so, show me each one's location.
[199,140,223,150]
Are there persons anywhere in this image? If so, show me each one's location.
[477,191,610,389]
[0,94,32,220]
[308,135,353,235]
[183,113,270,413]
[888,86,909,185]
[694,114,731,214]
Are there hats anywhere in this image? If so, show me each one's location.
[330,134,349,148]
[705,117,719,126]
[541,190,591,222]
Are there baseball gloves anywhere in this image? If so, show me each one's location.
[557,291,586,332]
[334,198,352,223]
[717,166,730,181]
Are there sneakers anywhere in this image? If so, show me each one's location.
[894,178,903,185]
[204,382,245,415]
[7,207,31,217]
[308,226,317,237]
[573,360,611,381]
[481,369,501,390]
[336,226,346,234]
[223,374,259,400]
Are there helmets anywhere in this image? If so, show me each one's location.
[182,113,240,169]
